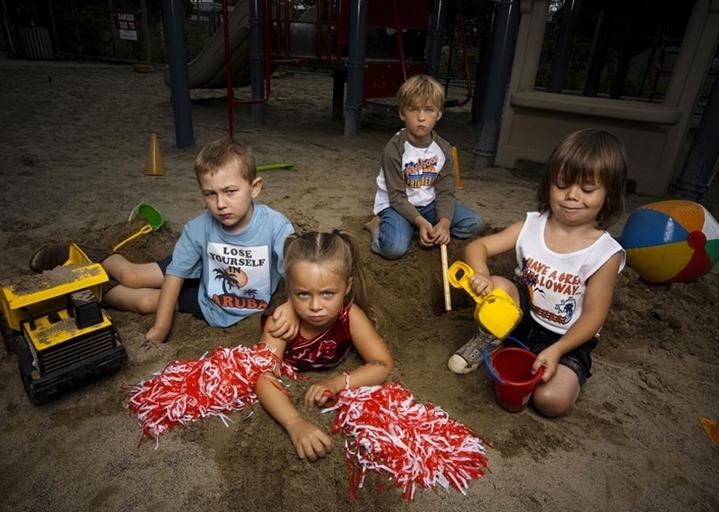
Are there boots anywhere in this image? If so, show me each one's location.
[29,243,120,296]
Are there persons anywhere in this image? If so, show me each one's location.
[363,74,485,260]
[253,226,392,461]
[446,129,629,419]
[26,136,296,344]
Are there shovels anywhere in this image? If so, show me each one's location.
[447,260,522,340]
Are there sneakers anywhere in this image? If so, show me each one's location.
[448,326,503,374]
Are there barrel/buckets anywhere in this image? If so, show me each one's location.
[482,337,546,413]
[127,201,165,234]
